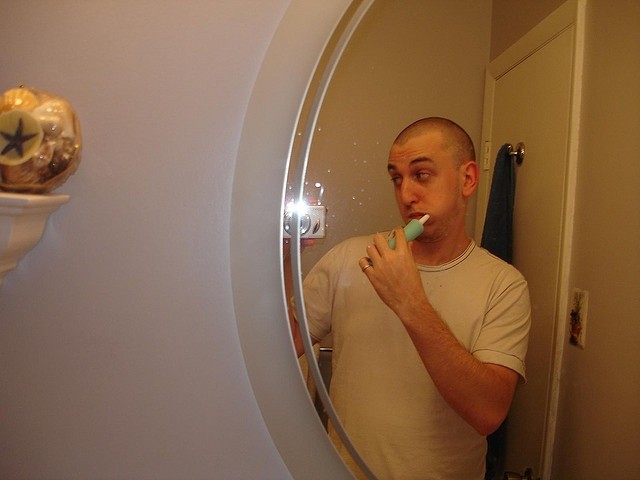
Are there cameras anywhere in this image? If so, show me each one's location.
[282,203,326,240]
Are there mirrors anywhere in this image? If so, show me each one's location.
[228,2,639,480]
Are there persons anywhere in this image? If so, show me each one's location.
[282,116,530,480]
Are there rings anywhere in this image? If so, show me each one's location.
[361,264,373,272]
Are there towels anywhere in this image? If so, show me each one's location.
[476,142,517,479]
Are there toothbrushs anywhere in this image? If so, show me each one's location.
[388,214,429,249]
